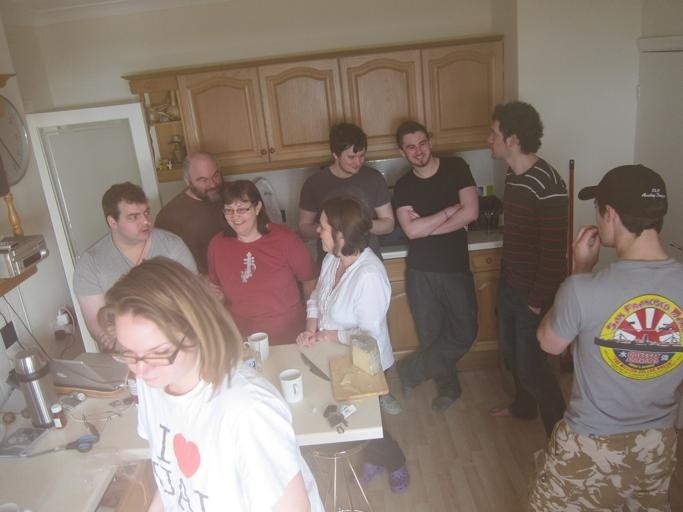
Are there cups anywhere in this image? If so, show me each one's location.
[243,332,269,359]
[280,368,303,403]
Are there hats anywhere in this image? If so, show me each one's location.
[578,165,668,218]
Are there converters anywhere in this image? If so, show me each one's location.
[55,330,66,341]
[56,313,70,326]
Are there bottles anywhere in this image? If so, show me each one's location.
[50,402,67,429]
[14,347,60,430]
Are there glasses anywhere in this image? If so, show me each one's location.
[110,334,190,366]
[219,202,254,215]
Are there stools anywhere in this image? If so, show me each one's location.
[309,441,373,512]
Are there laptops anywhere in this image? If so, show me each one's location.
[47,353,129,390]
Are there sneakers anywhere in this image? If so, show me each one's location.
[381,393,402,414]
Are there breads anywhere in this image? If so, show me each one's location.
[350,336,383,376]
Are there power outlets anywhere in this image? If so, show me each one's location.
[44,321,72,353]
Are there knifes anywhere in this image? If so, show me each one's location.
[300,352,329,381]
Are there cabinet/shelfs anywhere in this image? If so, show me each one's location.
[121,66,190,183]
[381,250,418,351]
[338,35,505,160]
[468,241,504,353]
[176,49,344,176]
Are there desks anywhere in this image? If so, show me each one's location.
[0,337,384,511]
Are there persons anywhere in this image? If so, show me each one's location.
[295,196,410,493]
[487,101,569,438]
[95,256,325,512]
[298,124,395,268]
[383,120,480,412]
[154,151,234,279]
[73,182,199,351]
[525,164,683,512]
[207,179,317,349]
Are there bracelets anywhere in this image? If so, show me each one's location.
[443,209,449,219]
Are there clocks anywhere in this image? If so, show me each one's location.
[0,95,31,187]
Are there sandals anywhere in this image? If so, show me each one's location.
[349,462,385,484]
[389,466,409,490]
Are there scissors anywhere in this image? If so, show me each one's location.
[27,434,101,460]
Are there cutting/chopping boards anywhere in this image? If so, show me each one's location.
[330,355,389,401]
